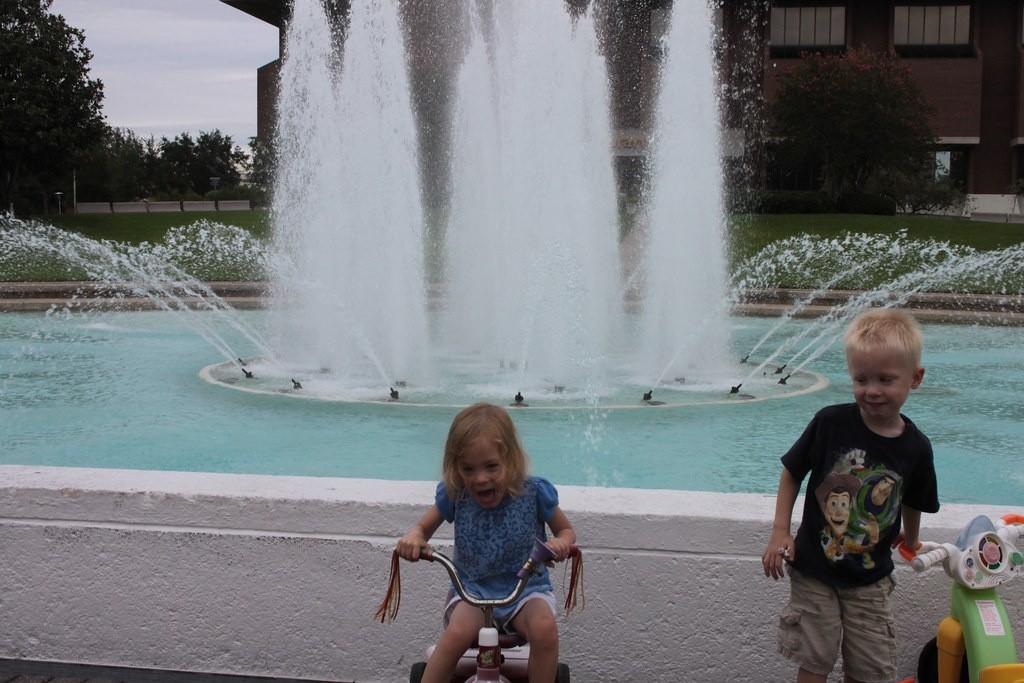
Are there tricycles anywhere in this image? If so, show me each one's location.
[374,536,589,682]
[891,514,1024,683]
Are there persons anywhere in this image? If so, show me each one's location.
[762,307,940,683]
[395,402,578,683]
